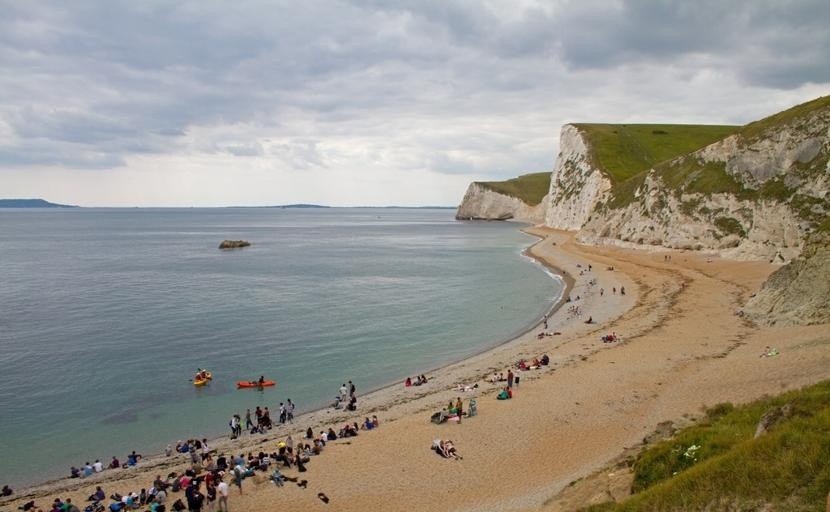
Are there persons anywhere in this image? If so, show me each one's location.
[600,288,605,296]
[406,375,427,386]
[493,370,512,398]
[540,353,550,366]
[70,450,141,478]
[2,484,105,511]
[602,332,617,343]
[519,358,528,371]
[216,436,293,495]
[165,438,214,473]
[543,315,548,329]
[259,376,264,383]
[294,415,378,471]
[532,357,541,366]
[334,381,356,412]
[620,285,626,295]
[229,399,294,439]
[613,286,617,295]
[440,397,462,460]
[195,370,206,380]
[126,470,228,511]
[587,315,592,323]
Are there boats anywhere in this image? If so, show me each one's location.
[193,372,212,385]
[236,381,277,386]
